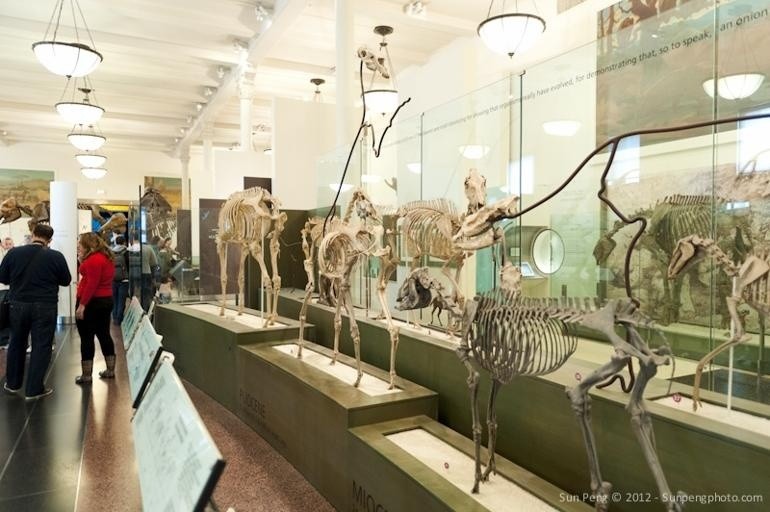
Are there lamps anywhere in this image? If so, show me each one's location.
[361,22,401,120]
[30,0,109,182]
[476,0,548,66]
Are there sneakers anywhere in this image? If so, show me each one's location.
[24,388,54,401]
[4,383,23,393]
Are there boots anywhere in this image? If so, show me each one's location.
[98,354,117,378]
[75,360,94,384]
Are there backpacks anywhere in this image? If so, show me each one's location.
[111,250,129,283]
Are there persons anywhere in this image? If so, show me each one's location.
[128,232,183,312]
[74,232,118,384]
[5,236,15,250]
[0,223,72,401]
[0,238,12,350]
[110,235,128,326]
